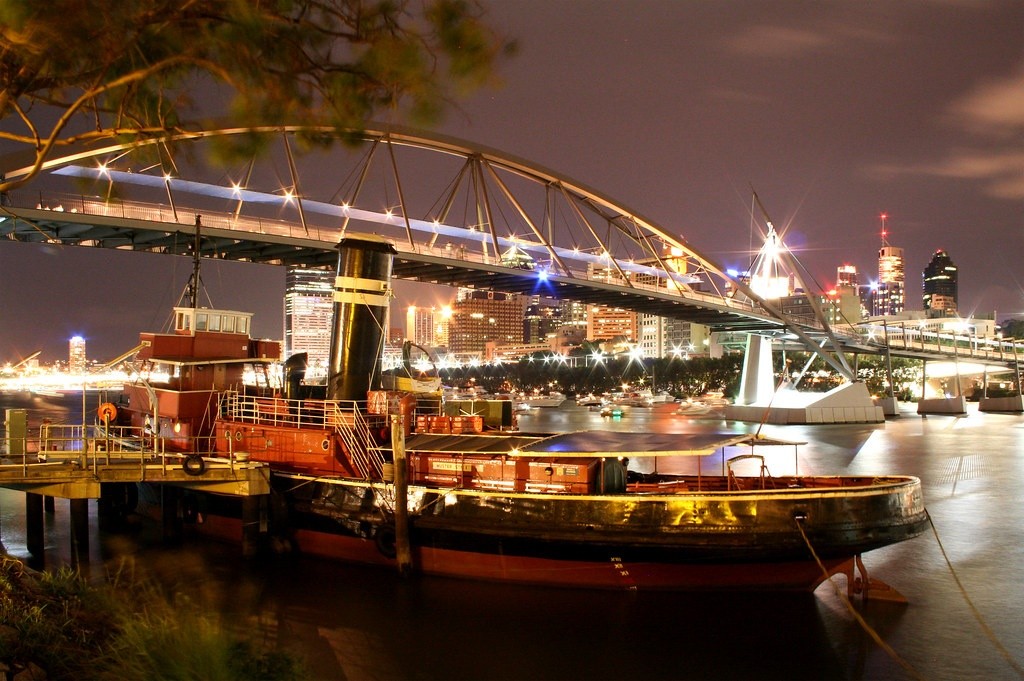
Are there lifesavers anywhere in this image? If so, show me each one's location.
[375,520,399,560]
[183,455,205,476]
[98,402,117,421]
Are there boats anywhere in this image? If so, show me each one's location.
[446,379,731,418]
[93,213,935,596]
